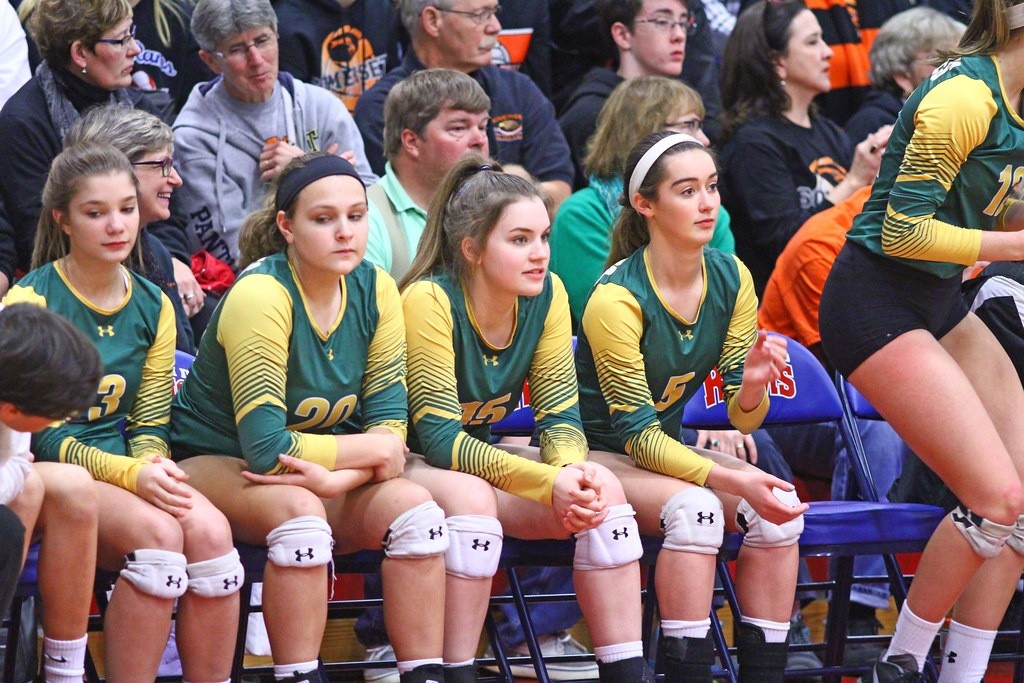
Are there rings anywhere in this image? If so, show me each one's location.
[711,439,719,447]
[266,160,269,170]
[186,294,194,301]
[736,444,744,450]
[869,144,878,154]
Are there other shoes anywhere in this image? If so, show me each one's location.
[787,619,823,680]
[399,662,511,683]
[858,648,938,683]
[823,615,885,665]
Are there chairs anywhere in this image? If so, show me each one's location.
[0,330,1024,683]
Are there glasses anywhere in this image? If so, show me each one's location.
[434,5,502,23]
[634,17,697,35]
[131,155,172,176]
[206,31,280,59]
[97,23,137,47]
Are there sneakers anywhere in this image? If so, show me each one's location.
[482,630,600,680]
[364,646,400,682]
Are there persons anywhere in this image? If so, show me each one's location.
[0,0,1024,683]
